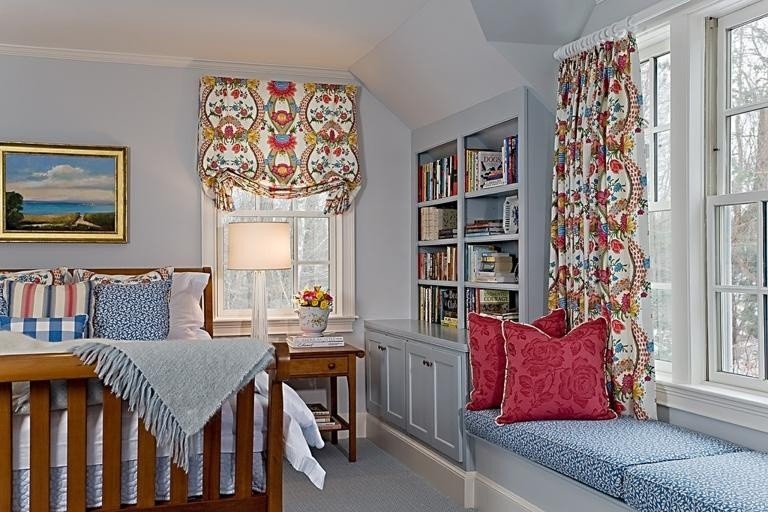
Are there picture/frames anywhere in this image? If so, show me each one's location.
[0,141,129,245]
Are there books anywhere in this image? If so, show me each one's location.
[304,404,342,431]
[420,207,457,242]
[418,154,458,204]
[285,335,344,348]
[465,287,519,330]
[465,136,517,193]
[419,285,458,330]
[419,246,457,281]
[465,219,505,237]
[464,243,518,284]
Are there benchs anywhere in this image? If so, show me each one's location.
[464,402,764,511]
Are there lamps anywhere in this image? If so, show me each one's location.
[227,221,293,343]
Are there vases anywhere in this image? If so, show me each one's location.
[293,304,333,337]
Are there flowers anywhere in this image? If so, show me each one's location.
[291,283,334,311]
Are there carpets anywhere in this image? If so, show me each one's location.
[281,436,468,512]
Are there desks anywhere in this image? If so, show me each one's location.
[289,342,366,462]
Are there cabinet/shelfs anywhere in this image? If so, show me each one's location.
[410,87,556,330]
[363,328,407,434]
[404,339,475,473]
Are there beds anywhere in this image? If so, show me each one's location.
[0,267,290,512]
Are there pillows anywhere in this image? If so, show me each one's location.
[0,314,89,342]
[72,265,174,341]
[92,279,172,341]
[0,278,92,339]
[493,319,617,428]
[466,309,567,411]
[0,266,68,294]
